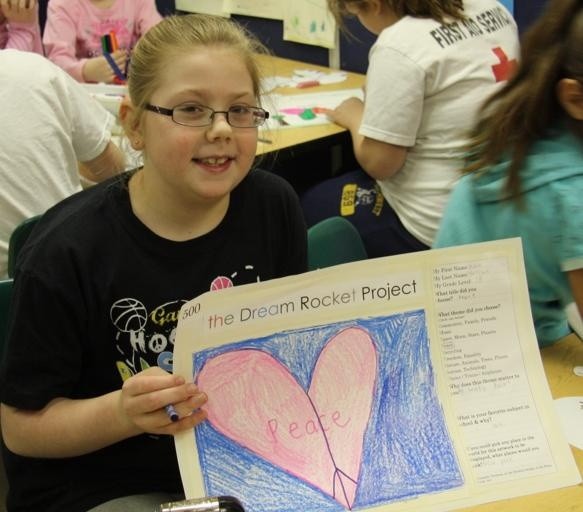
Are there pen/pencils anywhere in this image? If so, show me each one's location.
[102,31,117,55]
[165,405,178,422]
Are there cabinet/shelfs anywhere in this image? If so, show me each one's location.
[174,1,340,69]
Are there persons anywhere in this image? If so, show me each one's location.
[43,0,164,83]
[0,17,313,511]
[434,0,582,344]
[0,48,127,282]
[0,1,44,55]
[303,2,520,259]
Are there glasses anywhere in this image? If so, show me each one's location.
[144,103,269,127]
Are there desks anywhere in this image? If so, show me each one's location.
[75,35,366,189]
